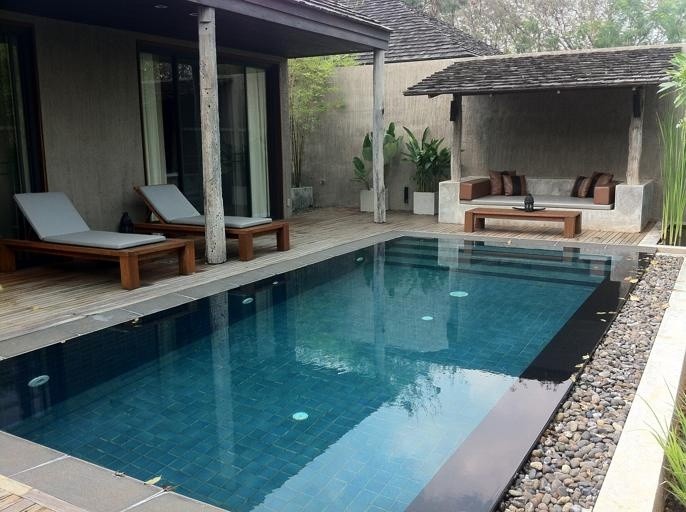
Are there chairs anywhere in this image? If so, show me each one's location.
[0,192,195,291]
[132,182,289,261]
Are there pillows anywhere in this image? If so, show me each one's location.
[489,168,521,196]
[569,171,614,197]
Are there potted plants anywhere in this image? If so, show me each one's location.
[349,121,400,212]
[400,125,451,216]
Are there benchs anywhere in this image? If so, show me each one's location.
[438,170,654,234]
